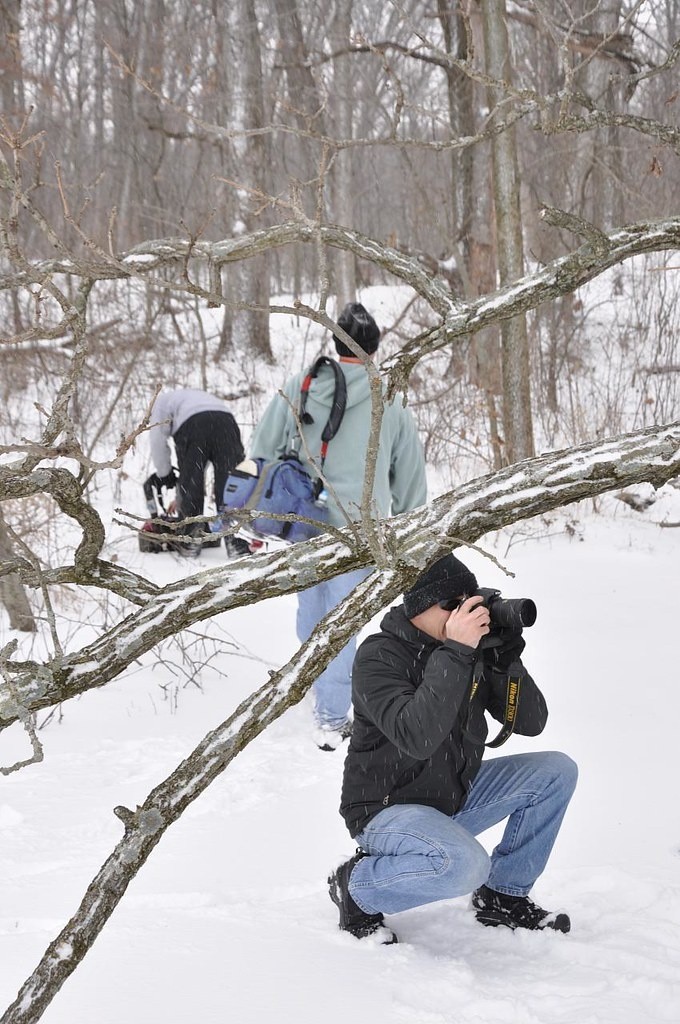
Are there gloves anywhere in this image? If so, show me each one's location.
[161,471,176,488]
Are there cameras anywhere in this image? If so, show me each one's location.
[468,587,537,628]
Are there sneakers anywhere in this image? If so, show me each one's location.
[328,846,399,945]
[470,932,570,933]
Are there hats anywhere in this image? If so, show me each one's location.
[332,303,381,357]
[402,552,479,619]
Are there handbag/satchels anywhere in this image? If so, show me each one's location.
[222,456,331,544]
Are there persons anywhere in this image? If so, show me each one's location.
[249,302,432,756]
[146,389,251,557]
[327,550,583,945]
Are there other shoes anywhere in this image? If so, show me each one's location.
[317,720,353,751]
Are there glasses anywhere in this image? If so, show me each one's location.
[438,599,461,611]
